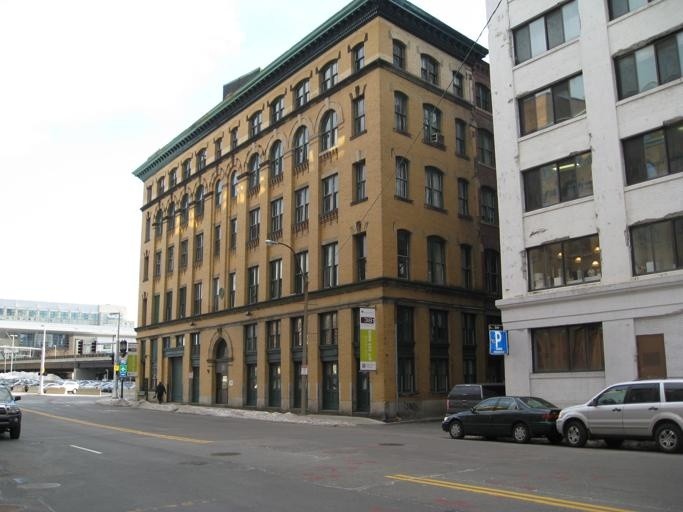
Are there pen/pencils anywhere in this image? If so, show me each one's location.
[447,380,505,418]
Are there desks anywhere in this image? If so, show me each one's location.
[52,344,57,359]
[37,323,47,395]
[10,333,18,374]
[263,236,309,415]
[108,311,122,400]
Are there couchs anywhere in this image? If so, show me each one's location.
[91,340,97,353]
[78,339,84,356]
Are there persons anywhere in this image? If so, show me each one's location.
[152,380,167,403]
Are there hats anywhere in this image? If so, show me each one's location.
[0,374,135,395]
[441,397,567,445]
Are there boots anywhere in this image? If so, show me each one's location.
[0,386,24,439]
[555,376,683,454]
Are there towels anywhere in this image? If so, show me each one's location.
[136,391,146,400]
